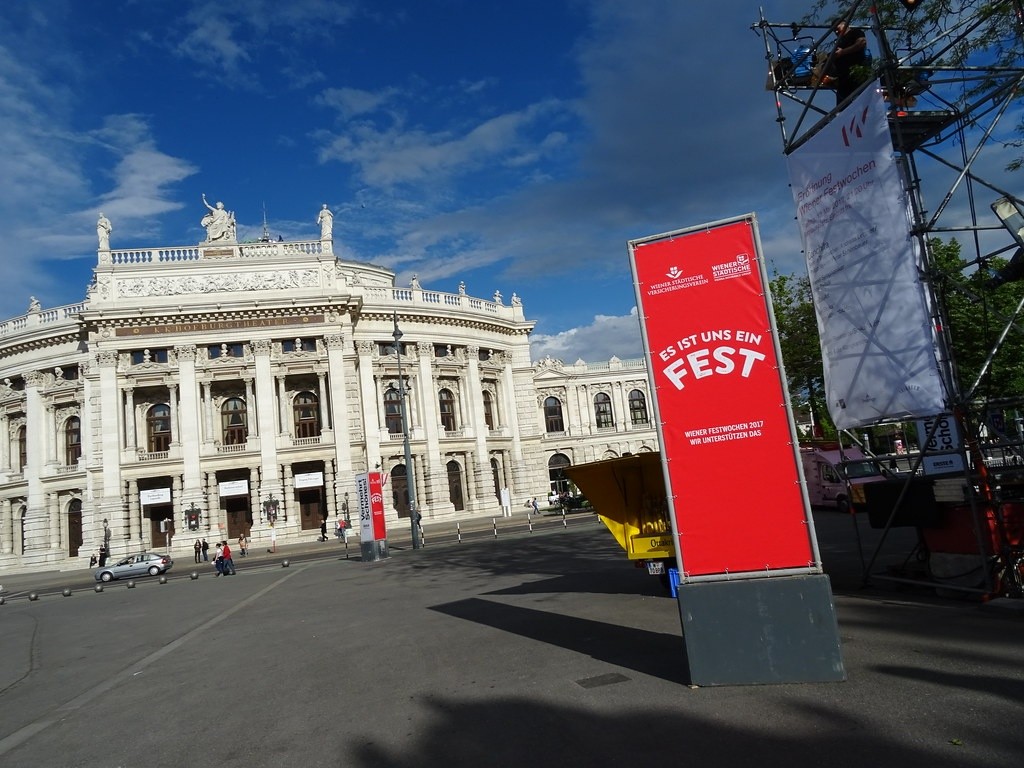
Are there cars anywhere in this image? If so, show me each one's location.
[94,552,177,584]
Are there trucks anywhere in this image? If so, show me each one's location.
[798,439,885,514]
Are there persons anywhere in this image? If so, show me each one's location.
[493,290,502,303]
[200,193,236,242]
[238,534,248,557]
[524,498,541,515]
[90,545,106,569]
[320,520,328,542]
[408,510,421,530]
[96,213,112,249]
[459,281,465,295]
[29,296,41,312]
[317,204,333,240]
[511,293,519,305]
[194,538,208,563]
[213,541,236,577]
[551,488,570,498]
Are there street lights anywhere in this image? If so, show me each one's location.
[393,309,420,550]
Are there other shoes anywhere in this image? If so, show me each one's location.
[217,573,224,577]
[322,540,325,542]
[326,537,328,541]
[232,572,236,575]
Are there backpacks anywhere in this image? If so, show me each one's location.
[532,501,535,506]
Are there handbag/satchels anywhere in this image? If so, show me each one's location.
[211,561,216,566]
